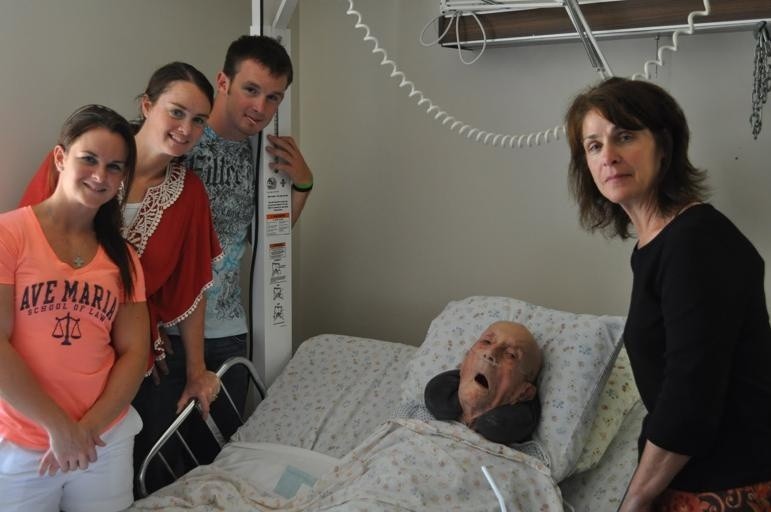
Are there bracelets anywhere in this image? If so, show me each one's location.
[293,183,314,192]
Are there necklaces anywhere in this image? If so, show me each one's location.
[46,200,94,269]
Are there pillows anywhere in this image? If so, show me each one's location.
[564,346,640,477]
[425,369,540,445]
[401,292,631,480]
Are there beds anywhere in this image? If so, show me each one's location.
[135,287,650,509]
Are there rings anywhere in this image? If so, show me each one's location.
[212,395,218,401]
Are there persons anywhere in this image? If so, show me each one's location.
[0,103,150,512]
[563,77,770,512]
[135,37,314,504]
[16,62,222,422]
[398,320,551,472]
